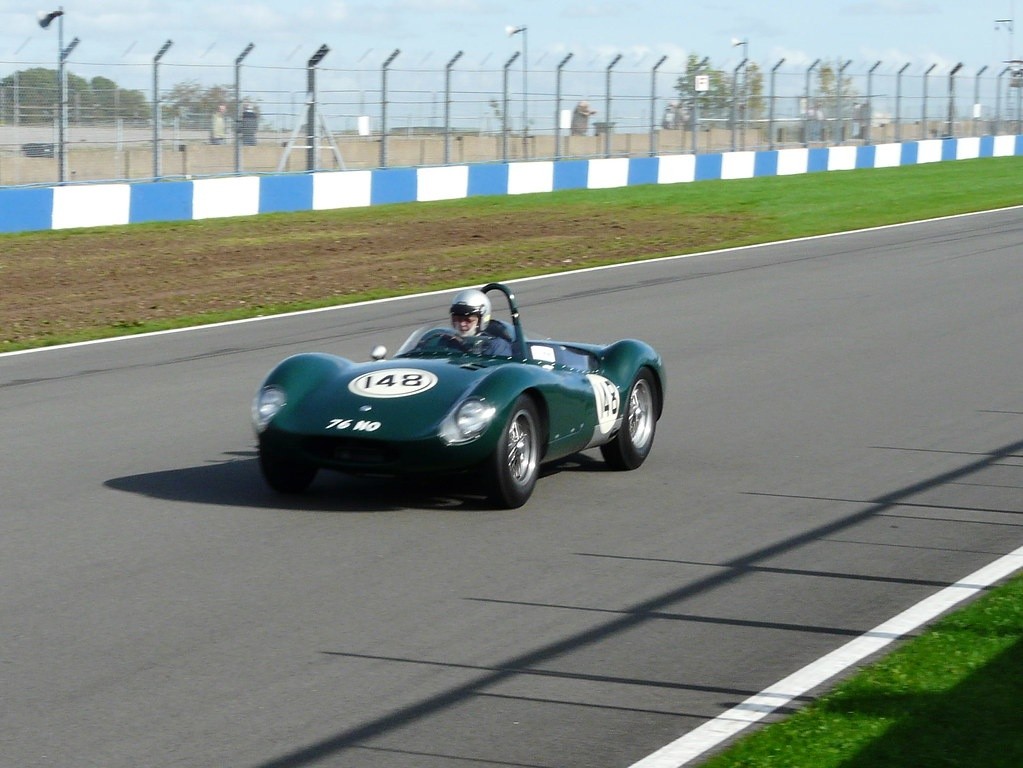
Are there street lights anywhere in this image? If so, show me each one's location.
[38,5,81,183]
[444,51,463,164]
[834,59,854,145]
[730,38,746,150]
[805,60,818,147]
[947,61,963,136]
[770,58,786,150]
[152,40,174,177]
[865,60,882,146]
[649,55,668,154]
[974,64,989,136]
[235,43,255,173]
[995,66,1014,136]
[605,54,623,157]
[381,48,401,167]
[691,56,709,155]
[555,52,574,157]
[503,52,520,159]
[921,63,937,140]
[895,62,911,143]
[506,25,529,135]
[307,44,330,172]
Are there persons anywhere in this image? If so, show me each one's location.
[436,289,511,355]
[238,103,258,146]
[210,105,227,145]
[662,102,696,131]
[852,106,872,139]
[570,102,597,136]
[808,105,826,142]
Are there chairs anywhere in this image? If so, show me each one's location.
[489,319,512,343]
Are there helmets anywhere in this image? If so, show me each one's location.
[449,290,491,332]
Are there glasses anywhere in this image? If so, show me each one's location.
[451,314,479,323]
